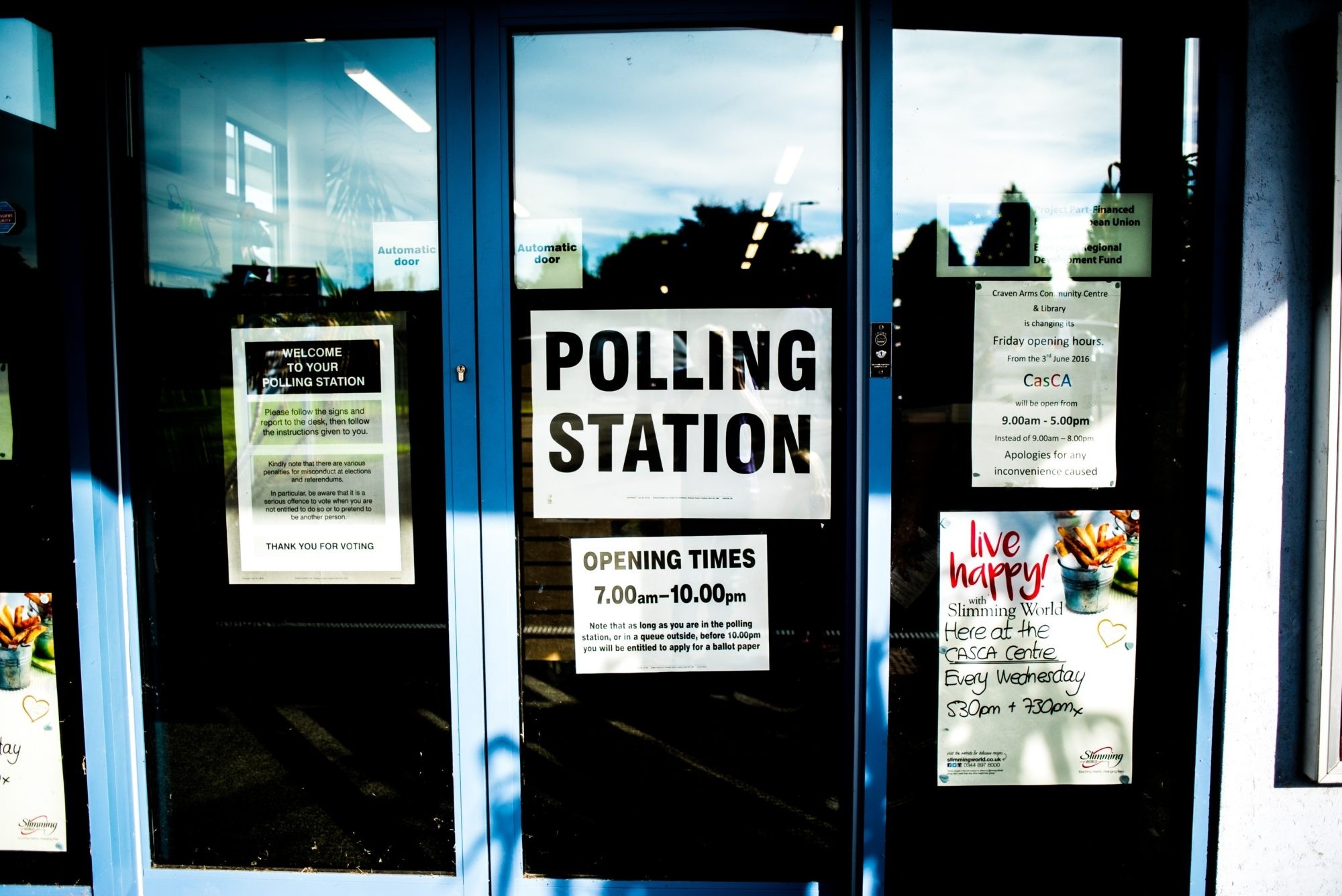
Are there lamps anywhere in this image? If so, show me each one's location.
[343,49,434,134]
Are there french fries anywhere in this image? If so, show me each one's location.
[1109,509,1139,536]
[0,605,47,646]
[24,592,53,620]
[1054,523,1132,568]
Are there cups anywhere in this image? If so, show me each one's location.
[0,643,33,690]
[1058,558,1117,614]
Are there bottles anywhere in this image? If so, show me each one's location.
[34,620,54,659]
[1118,536,1139,580]
